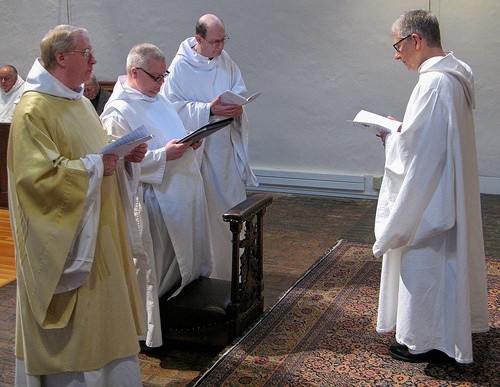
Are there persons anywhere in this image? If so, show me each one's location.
[373,9,491,365]
[6,24,148,387]
[159,14,262,282]
[0,63,32,123]
[98,43,211,301]
[82,73,112,116]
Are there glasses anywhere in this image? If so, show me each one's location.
[57,48,94,58]
[201,34,230,45]
[393,33,422,52]
[136,67,170,82]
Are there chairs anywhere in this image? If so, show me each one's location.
[165,192,274,356]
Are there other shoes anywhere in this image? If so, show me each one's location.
[388,342,455,362]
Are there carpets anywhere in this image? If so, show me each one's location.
[186,237,500,387]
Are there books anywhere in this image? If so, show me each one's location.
[215,89,261,108]
[99,124,153,161]
[346,108,403,138]
[174,117,235,149]
